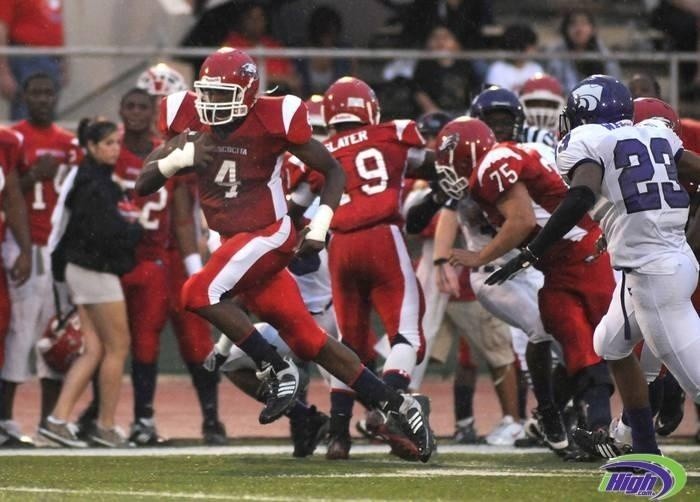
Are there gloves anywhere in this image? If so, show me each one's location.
[484,245,539,287]
[287,204,336,276]
[154,131,217,180]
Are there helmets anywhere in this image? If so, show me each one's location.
[557,74,634,138]
[39,312,87,376]
[155,90,210,134]
[192,46,259,126]
[137,63,188,96]
[302,74,382,128]
[415,86,526,200]
[631,95,682,137]
[678,118,700,155]
[520,73,566,130]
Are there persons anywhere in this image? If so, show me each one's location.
[2,0,699,474]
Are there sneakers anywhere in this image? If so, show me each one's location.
[202,419,229,445]
[86,411,170,448]
[515,372,686,475]
[291,404,332,457]
[453,420,475,444]
[326,436,352,459]
[255,355,309,425]
[38,416,87,449]
[358,392,437,462]
[0,418,35,449]
[485,417,521,446]
